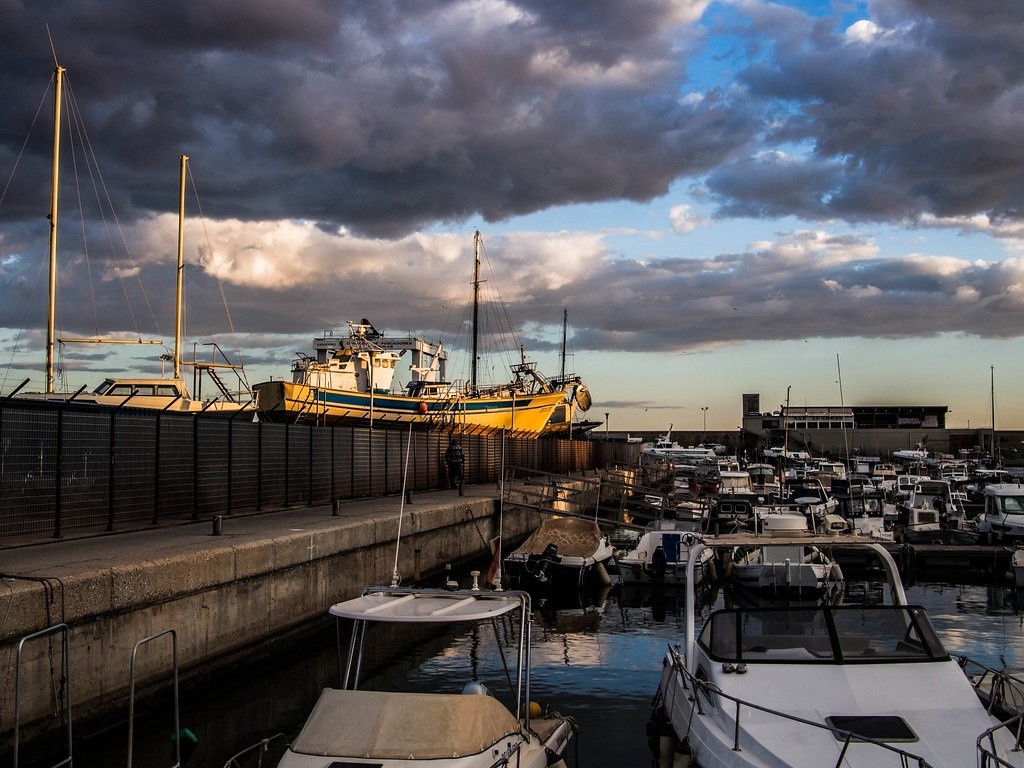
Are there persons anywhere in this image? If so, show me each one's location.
[922,435,929,444]
[445,439,465,490]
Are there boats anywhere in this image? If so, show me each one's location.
[227,417,579,767]
[1007,549,1024,584]
[627,357,1024,547]
[721,543,843,588]
[501,513,617,588]
[644,503,1024,768]
[623,529,718,598]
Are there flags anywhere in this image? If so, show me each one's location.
[488,544,501,586]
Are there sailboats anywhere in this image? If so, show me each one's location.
[0,63,261,416]
[249,229,605,442]
